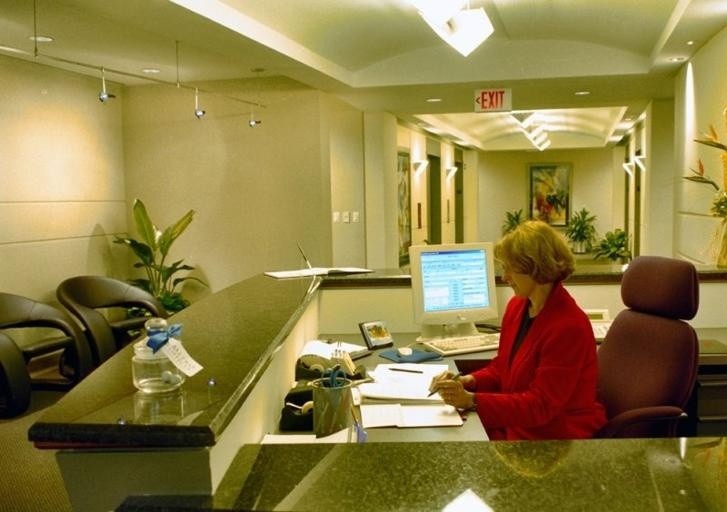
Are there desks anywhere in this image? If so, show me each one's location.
[271,327,727,444]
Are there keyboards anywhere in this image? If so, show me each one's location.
[423,332,500,357]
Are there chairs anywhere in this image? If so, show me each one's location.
[595,255,699,438]
[0,292,93,420]
[56,275,171,364]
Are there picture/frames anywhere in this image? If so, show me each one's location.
[528,160,573,231]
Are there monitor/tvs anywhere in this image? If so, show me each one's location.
[409,242,499,342]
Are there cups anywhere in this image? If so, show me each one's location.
[312,378,349,437]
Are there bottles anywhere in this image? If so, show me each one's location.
[131,392,187,424]
[130,318,188,392]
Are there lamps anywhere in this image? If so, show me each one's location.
[446,167,458,179]
[634,156,646,171]
[98,39,266,128]
[408,0,496,58]
[509,112,551,152]
[414,161,429,177]
[622,163,633,176]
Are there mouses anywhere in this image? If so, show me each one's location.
[398,347,413,358]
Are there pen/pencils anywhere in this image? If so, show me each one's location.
[389,367,423,373]
[428,372,463,397]
[297,242,311,269]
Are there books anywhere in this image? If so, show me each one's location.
[300,340,371,364]
[360,403,462,431]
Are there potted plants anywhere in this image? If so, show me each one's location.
[592,228,632,265]
[565,207,598,253]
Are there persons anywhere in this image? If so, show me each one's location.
[429,221,607,440]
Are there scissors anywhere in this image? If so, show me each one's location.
[321,370,347,387]
